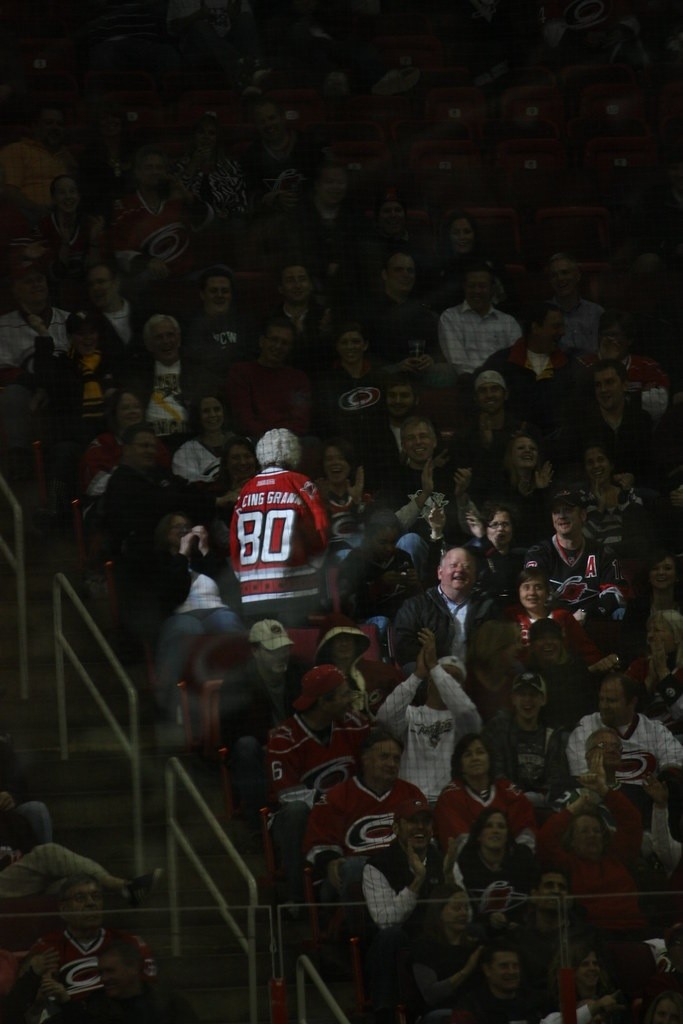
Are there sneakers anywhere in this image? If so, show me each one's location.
[120,868,162,907]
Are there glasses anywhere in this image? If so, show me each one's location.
[60,891,103,903]
[549,270,576,279]
[487,520,511,528]
[131,442,156,450]
[670,935,683,946]
[264,335,294,346]
[170,523,191,529]
[329,634,359,641]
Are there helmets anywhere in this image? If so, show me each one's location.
[256,428,303,470]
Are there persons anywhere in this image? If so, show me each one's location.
[2,71,681,1024]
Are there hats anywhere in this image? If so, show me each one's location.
[512,672,546,695]
[248,618,296,651]
[13,260,48,285]
[361,731,405,754]
[394,799,433,822]
[551,490,583,507]
[436,655,468,681]
[474,370,506,391]
[665,922,683,947]
[528,618,562,641]
[292,664,347,711]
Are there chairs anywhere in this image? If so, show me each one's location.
[1,443,443,1018]
